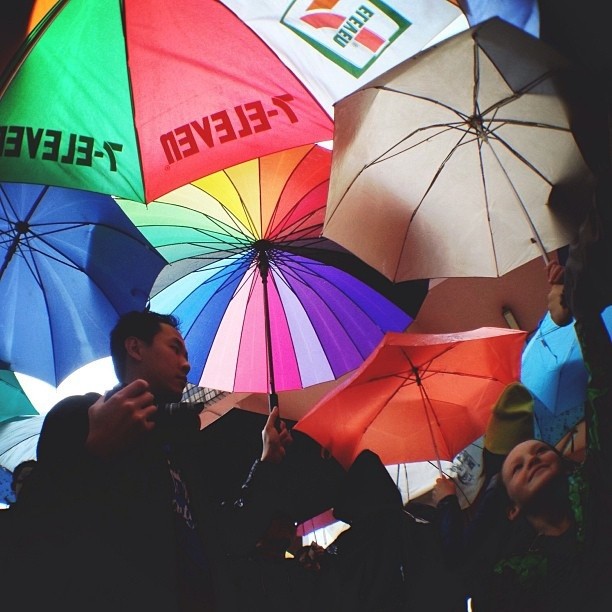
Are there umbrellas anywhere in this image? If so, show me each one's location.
[407,249,562,340]
[318,15,592,289]
[0,1,465,203]
[1,181,170,387]
[519,302,612,414]
[293,327,528,472]
[112,142,432,394]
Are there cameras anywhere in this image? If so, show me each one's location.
[102,383,204,443]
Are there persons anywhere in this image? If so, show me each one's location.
[547,204,612,327]
[431,383,535,563]
[478,260,611,612]
[1,311,293,612]
[305,449,432,610]
[12,459,39,491]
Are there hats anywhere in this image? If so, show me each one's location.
[484,382,534,456]
